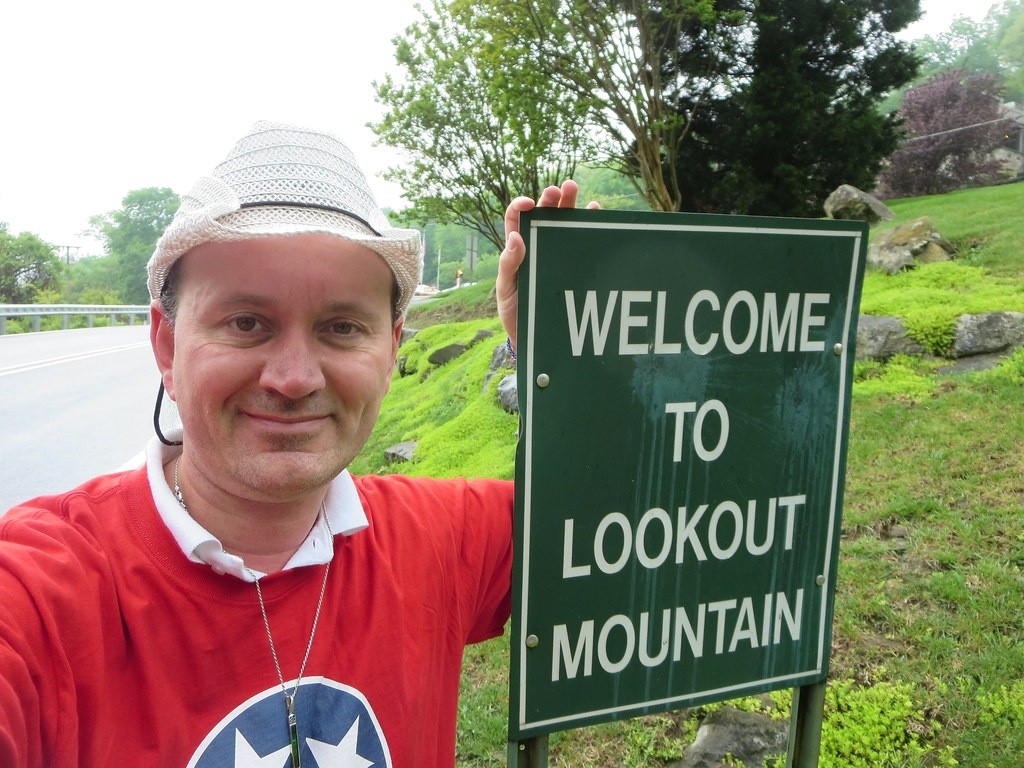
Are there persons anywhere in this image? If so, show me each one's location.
[0,121,604,768]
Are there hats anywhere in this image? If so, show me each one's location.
[147,118,425,317]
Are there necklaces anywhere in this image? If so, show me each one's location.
[173,454,334,768]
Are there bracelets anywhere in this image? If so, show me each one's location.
[506,337,517,361]
